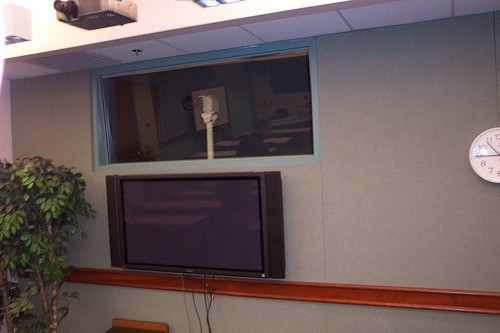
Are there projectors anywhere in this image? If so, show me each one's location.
[53,0,138,31]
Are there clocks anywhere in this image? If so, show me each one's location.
[467,126,500,184]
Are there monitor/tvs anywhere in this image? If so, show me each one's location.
[105,174,286,280]
